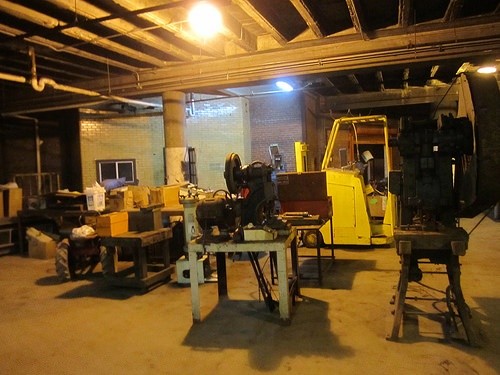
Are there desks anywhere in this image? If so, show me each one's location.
[388,227,477,348]
[187,228,299,326]
[269,216,334,286]
[102,227,173,288]
[16,208,184,255]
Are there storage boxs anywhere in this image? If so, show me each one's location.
[0,183,179,260]
[275,171,327,201]
[280,196,333,218]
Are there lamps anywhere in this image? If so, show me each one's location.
[276,78,296,91]
[477,55,497,74]
